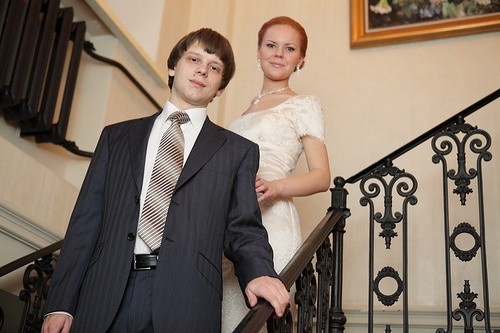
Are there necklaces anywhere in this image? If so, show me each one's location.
[254,86,289,105]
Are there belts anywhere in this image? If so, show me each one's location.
[131,254,158,270]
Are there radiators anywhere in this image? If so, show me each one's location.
[0,0,162,156]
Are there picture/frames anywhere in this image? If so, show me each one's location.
[349,0,500,49]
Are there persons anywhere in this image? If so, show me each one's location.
[39,28,289,333]
[220,16,331,333]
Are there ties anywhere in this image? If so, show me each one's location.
[138,111,191,251]
